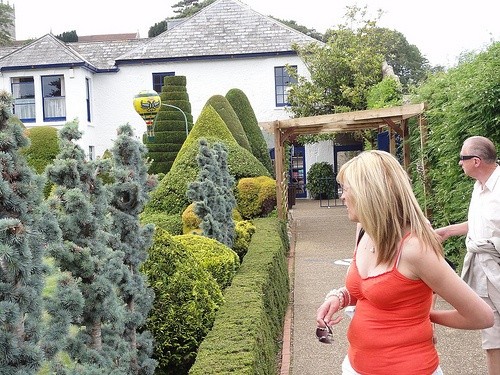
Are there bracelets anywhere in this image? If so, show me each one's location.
[325,289,344,311]
[338,288,346,308]
[342,286,351,307]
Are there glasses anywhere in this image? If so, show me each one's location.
[460,155,482,161]
[315,321,334,344]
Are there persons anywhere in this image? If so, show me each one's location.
[315,147,494,375]
[435,135,500,375]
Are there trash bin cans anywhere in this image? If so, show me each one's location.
[287,184,297,210]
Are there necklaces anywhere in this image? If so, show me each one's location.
[362,235,379,253]
